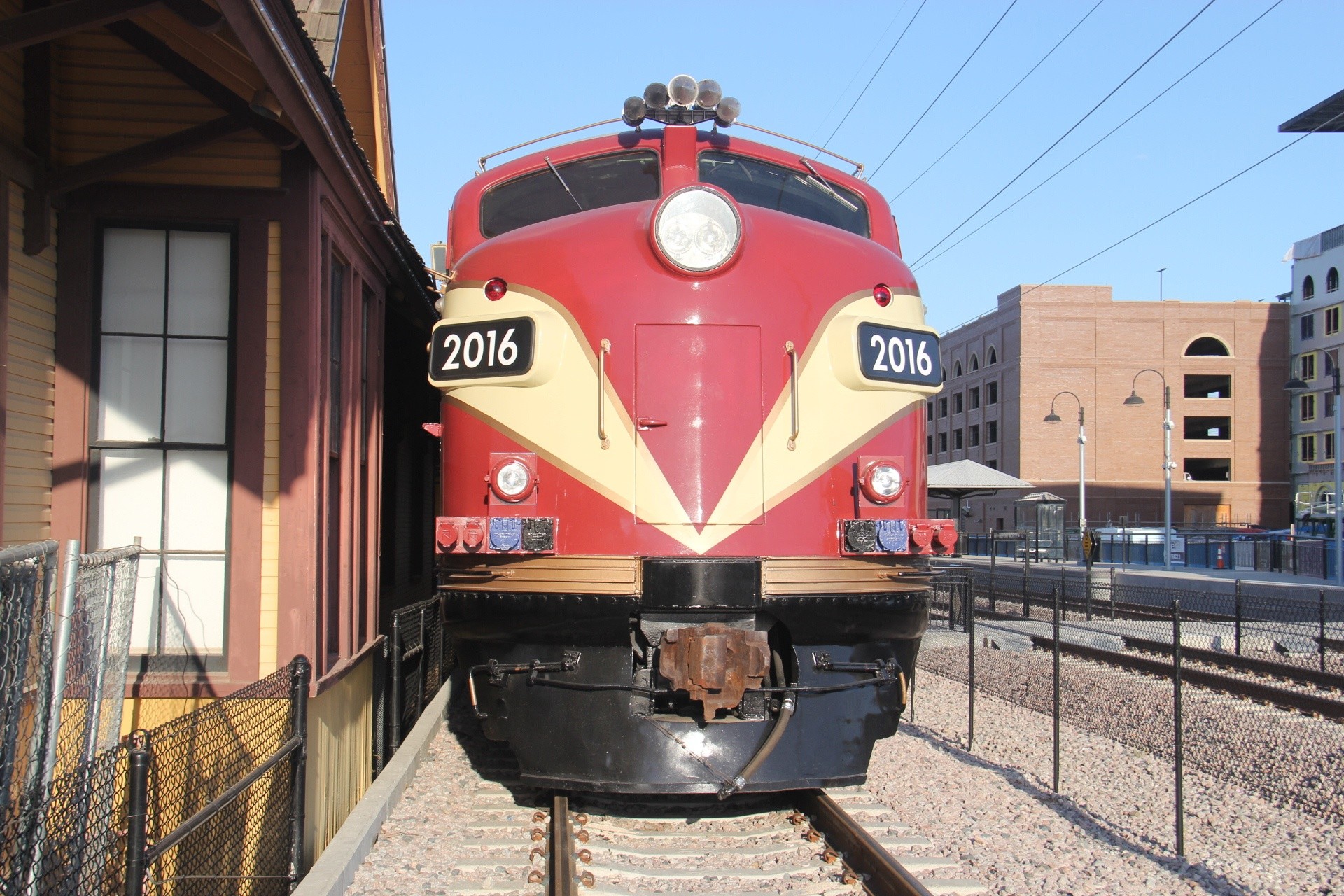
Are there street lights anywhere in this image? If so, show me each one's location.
[1281,348,1343,586]
[1156,268,1167,300]
[1044,391,1088,566]
[1123,369,1177,572]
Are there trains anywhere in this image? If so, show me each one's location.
[421,76,959,800]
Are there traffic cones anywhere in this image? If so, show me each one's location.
[1213,544,1228,569]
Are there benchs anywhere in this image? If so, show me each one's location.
[1018,531,1055,563]
